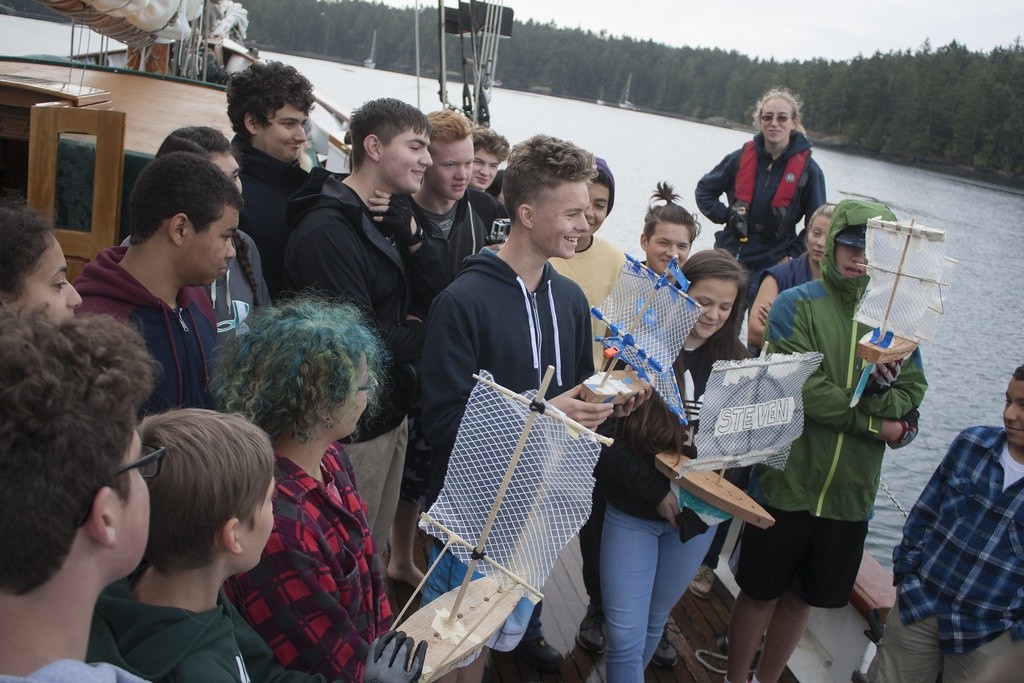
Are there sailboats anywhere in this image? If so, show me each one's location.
[618,73,640,111]
[363,29,377,68]
[597,85,607,105]
[656,341,825,528]
[856,216,959,363]
[388,363,615,683]
[578,253,705,427]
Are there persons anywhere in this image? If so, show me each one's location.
[688,199,928,683]
[694,85,826,361]
[0,125,429,682]
[279,97,510,590]
[867,361,1024,683]
[417,133,756,683]
[226,60,316,304]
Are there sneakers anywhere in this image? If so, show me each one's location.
[690,562,715,600]
[516,635,563,671]
[651,621,682,668]
[576,603,607,655]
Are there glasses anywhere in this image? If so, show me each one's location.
[352,373,379,402]
[74,443,165,527]
[759,114,792,122]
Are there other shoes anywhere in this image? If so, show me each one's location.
[717,632,767,669]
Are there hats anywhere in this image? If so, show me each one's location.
[838,222,869,249]
[595,157,614,212]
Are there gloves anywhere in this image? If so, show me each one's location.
[863,608,886,644]
[363,630,428,683]
